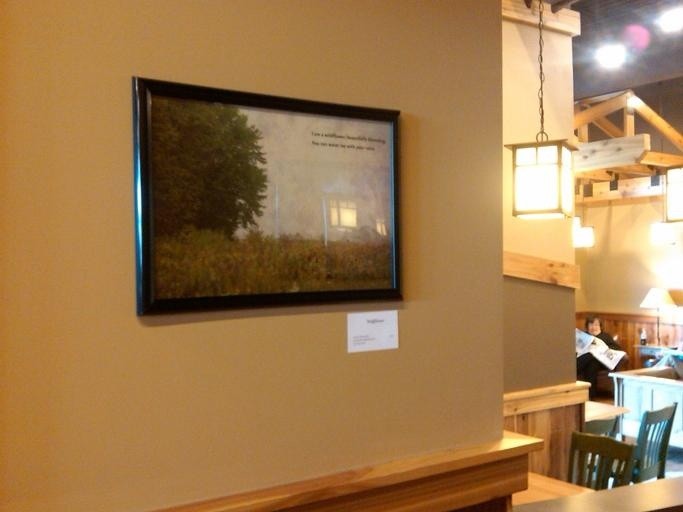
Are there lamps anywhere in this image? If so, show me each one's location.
[504,0,581,220]
[639,287,677,346]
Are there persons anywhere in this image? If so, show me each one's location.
[576,314,629,401]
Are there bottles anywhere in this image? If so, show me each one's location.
[640,330,647,346]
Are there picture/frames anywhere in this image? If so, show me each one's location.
[131,74,404,316]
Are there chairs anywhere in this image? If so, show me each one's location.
[568,401,678,491]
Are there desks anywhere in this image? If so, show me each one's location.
[633,344,677,369]
[512,472,594,507]
